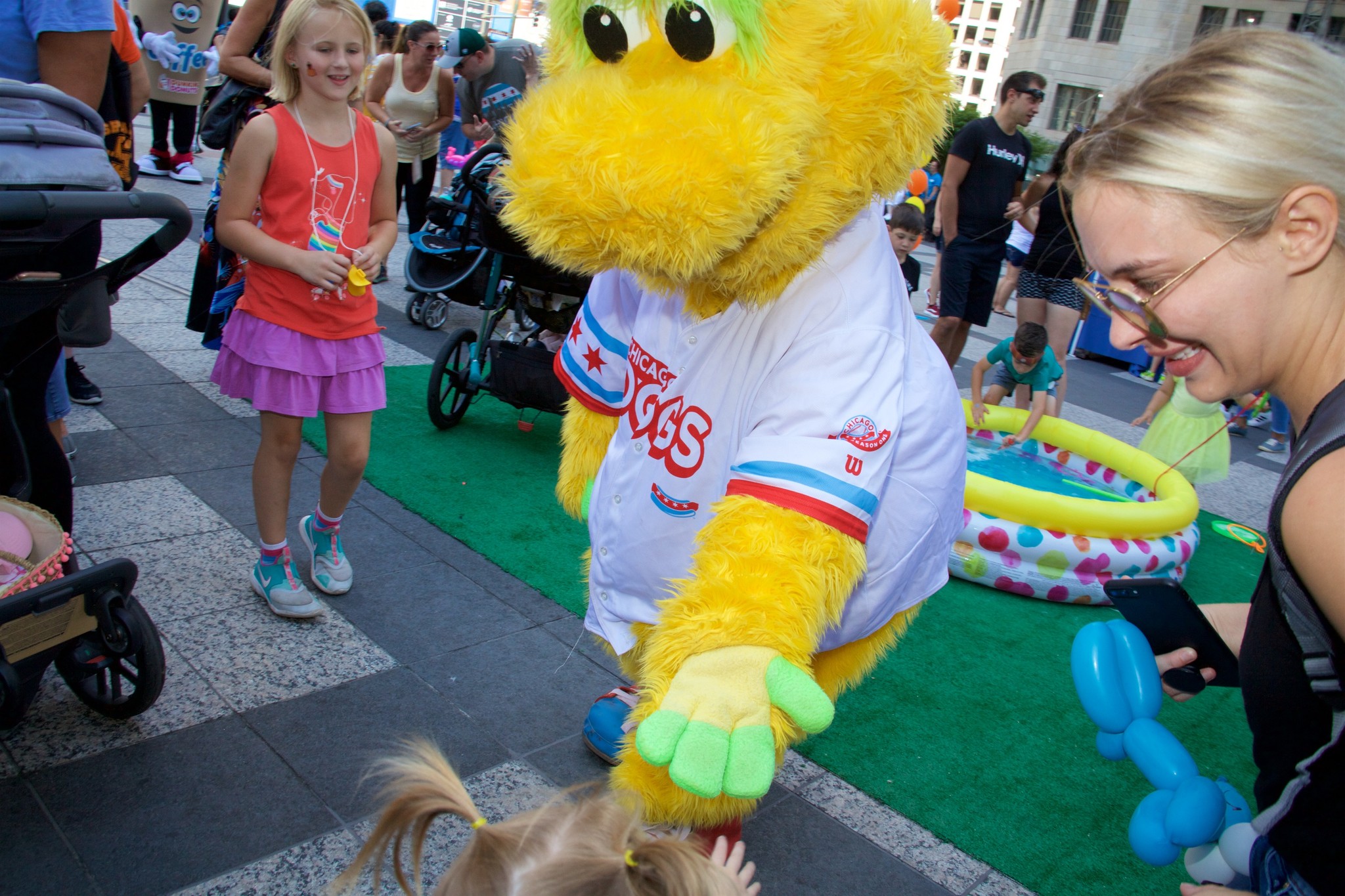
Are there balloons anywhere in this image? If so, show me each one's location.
[1070,620,1251,865]
[910,234,922,251]
[905,197,925,213]
[908,167,928,196]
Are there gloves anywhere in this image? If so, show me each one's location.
[141,31,180,69]
[202,45,219,76]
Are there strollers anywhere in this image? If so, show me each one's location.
[0,77,191,734]
[401,143,593,427]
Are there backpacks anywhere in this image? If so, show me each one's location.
[99,42,139,192]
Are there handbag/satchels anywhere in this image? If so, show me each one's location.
[200,73,266,151]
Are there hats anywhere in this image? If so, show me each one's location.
[437,28,486,69]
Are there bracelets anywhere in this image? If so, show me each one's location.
[385,118,392,127]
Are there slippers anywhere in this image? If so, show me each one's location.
[991,308,1015,318]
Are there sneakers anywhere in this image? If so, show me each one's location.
[1225,422,1247,437]
[299,508,353,595]
[1257,437,1286,453]
[1248,412,1273,426]
[66,362,103,404]
[1140,371,1156,382]
[1157,374,1166,385]
[925,287,931,304]
[252,544,324,620]
[924,305,940,319]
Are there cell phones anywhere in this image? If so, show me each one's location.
[405,122,422,130]
[1103,577,1241,687]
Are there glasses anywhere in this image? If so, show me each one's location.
[1017,88,1044,101]
[457,56,475,69]
[1073,124,1091,133]
[1072,230,1247,341]
[414,41,442,51]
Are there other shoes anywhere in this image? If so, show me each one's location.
[67,460,77,487]
[61,433,77,459]
[137,155,170,176]
[373,264,388,284]
[168,162,204,183]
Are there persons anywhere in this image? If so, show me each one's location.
[992,174,1055,318]
[924,189,946,317]
[1219,395,1272,426]
[1064,23,1345,896]
[869,160,941,242]
[340,736,760,895]
[62,0,149,405]
[126,18,220,181]
[1227,387,1288,452]
[1131,363,1272,490]
[362,1,455,293]
[0,0,116,482]
[1016,128,1090,415]
[929,69,1047,368]
[435,26,541,199]
[196,10,251,136]
[1139,354,1167,385]
[885,202,925,303]
[971,322,1064,450]
[211,0,397,617]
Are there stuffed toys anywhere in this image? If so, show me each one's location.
[486,0,969,848]
